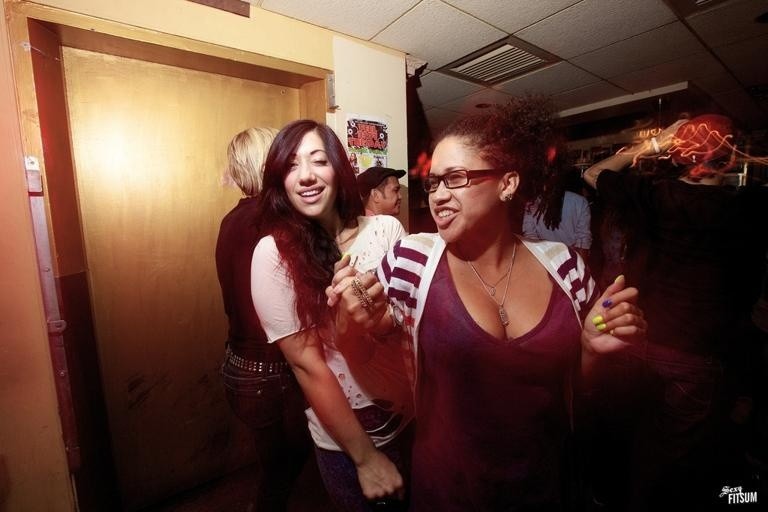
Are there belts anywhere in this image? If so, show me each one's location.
[228,353,288,374]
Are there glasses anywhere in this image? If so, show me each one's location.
[424,169,497,193]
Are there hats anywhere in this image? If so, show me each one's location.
[671,114,742,164]
[357,167,406,190]
